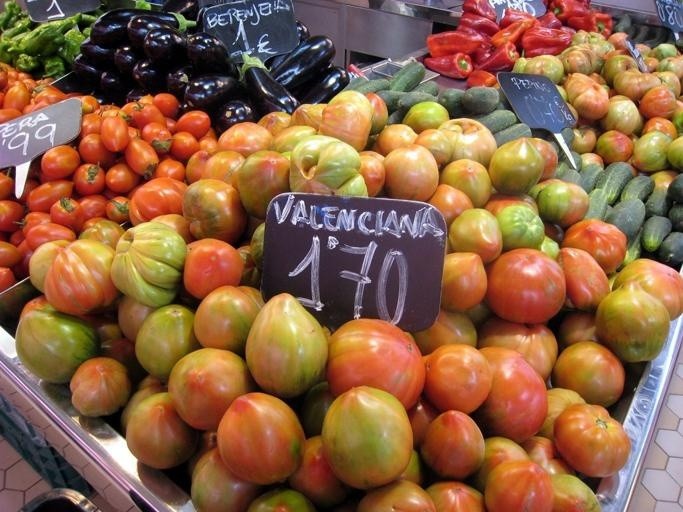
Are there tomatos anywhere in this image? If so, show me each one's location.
[0,29,683,512]
